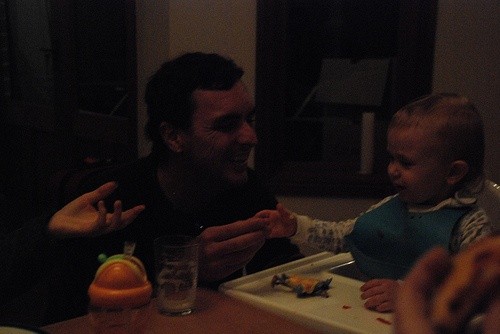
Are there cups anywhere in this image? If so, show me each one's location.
[87,242,152,334]
[155,235,199,316]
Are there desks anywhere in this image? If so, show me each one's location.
[34,246,397,334]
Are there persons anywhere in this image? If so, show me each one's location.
[82,51,272,283]
[396,237,500,334]
[253,93,500,312]
[1,181,146,285]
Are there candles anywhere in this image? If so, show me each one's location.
[360,110,377,172]
